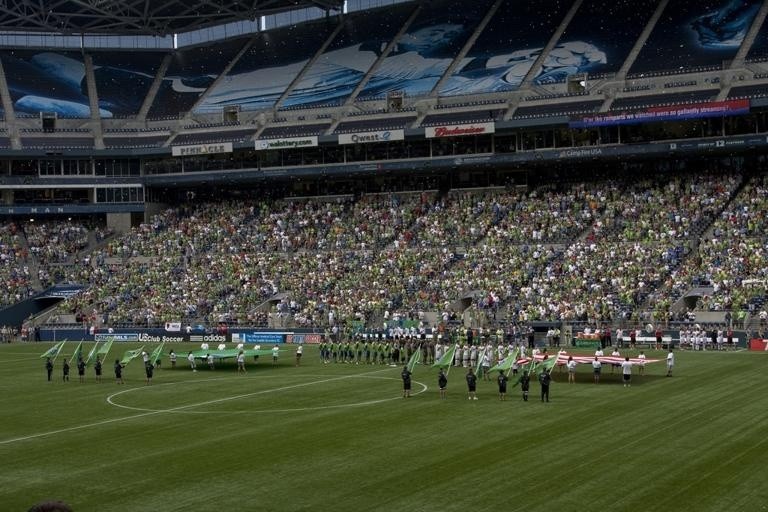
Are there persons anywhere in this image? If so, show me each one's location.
[172,341,303,372]
[758,322,765,339]
[44,350,161,386]
[595,324,664,352]
[746,325,753,352]
[73,175,768,329]
[402,365,552,400]
[557,346,674,386]
[318,321,436,367]
[680,326,735,351]
[564,330,572,347]
[432,323,560,381]
[1,217,117,343]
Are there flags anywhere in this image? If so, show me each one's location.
[120,345,144,366]
[95,339,112,356]
[40,340,63,360]
[432,345,454,369]
[524,361,535,374]
[535,356,556,376]
[70,342,83,363]
[474,353,483,380]
[487,352,516,374]
[517,354,659,367]
[84,343,98,364]
[407,348,420,372]
[150,345,162,365]
